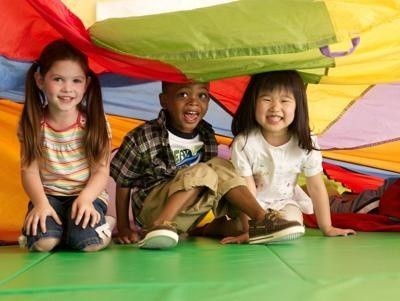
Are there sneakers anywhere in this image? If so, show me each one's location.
[137,221,179,250]
[247,208,306,245]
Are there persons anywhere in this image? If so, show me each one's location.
[187,70,358,247]
[110,78,305,251]
[16,37,117,255]
